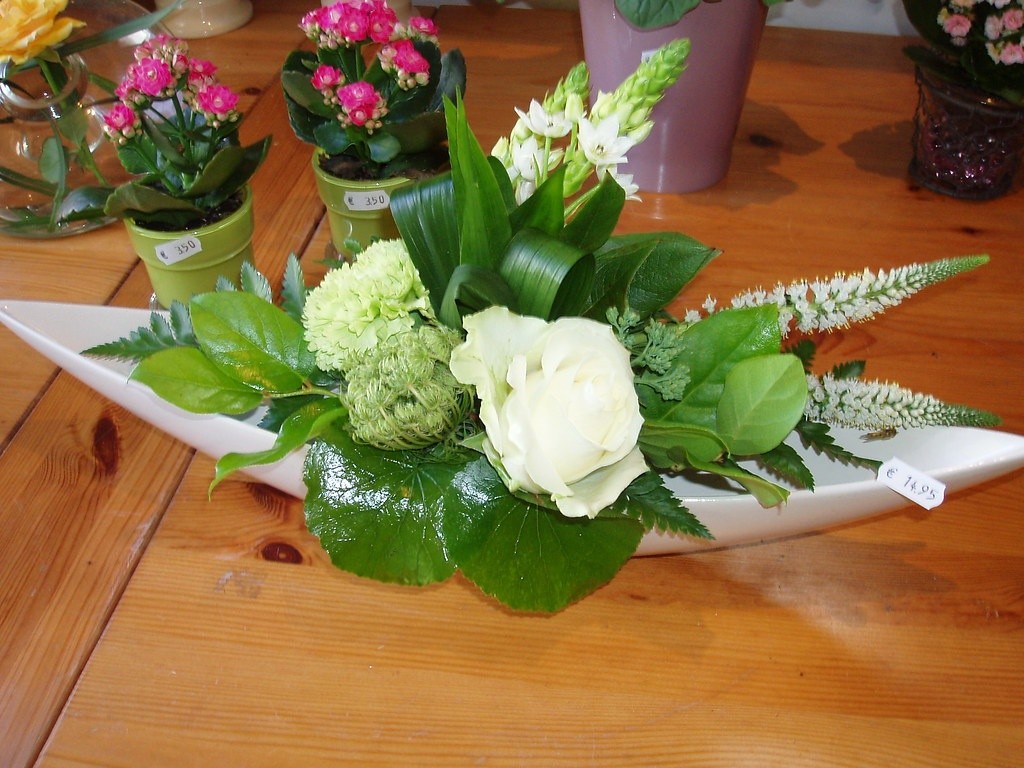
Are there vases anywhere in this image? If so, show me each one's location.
[321,1,412,28]
[576,0,771,192]
[1,0,175,237]
[901,42,1024,200]
[156,0,255,38]
[123,185,255,308]
[311,144,449,263]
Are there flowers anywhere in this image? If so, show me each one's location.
[78,40,1011,612]
[903,0,1024,102]
[281,1,467,180]
[0,0,182,227]
[102,32,273,222]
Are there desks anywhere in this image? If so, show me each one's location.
[0,0,1024,767]
